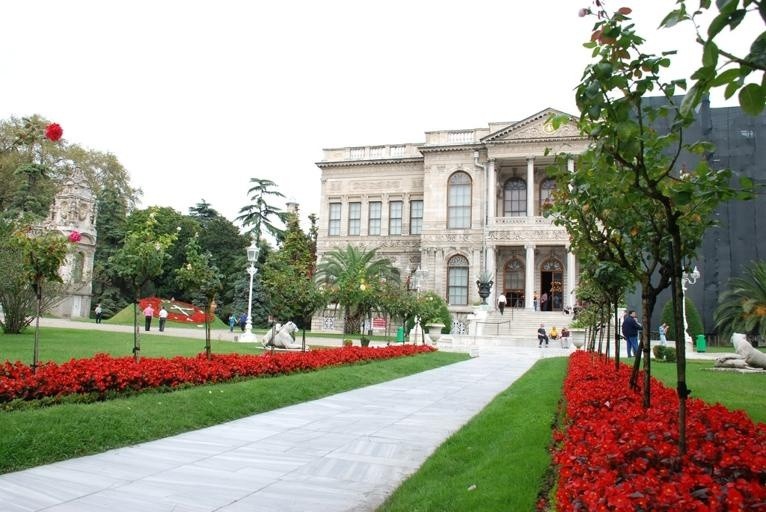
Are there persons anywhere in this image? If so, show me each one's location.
[498,292,508,316]
[143,303,153,331]
[95,303,102,324]
[622,310,644,358]
[549,325,559,340]
[561,327,570,348]
[541,289,549,311]
[538,323,548,348]
[633,316,641,356]
[238,311,248,332]
[659,321,670,347]
[158,306,168,331]
[228,312,238,333]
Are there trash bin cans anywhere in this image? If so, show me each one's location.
[695,335,706,352]
[397,326,405,342]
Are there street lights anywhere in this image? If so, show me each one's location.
[233,239,261,343]
[668,259,701,358]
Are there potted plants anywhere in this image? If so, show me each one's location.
[425,316,446,349]
[567,319,586,350]
